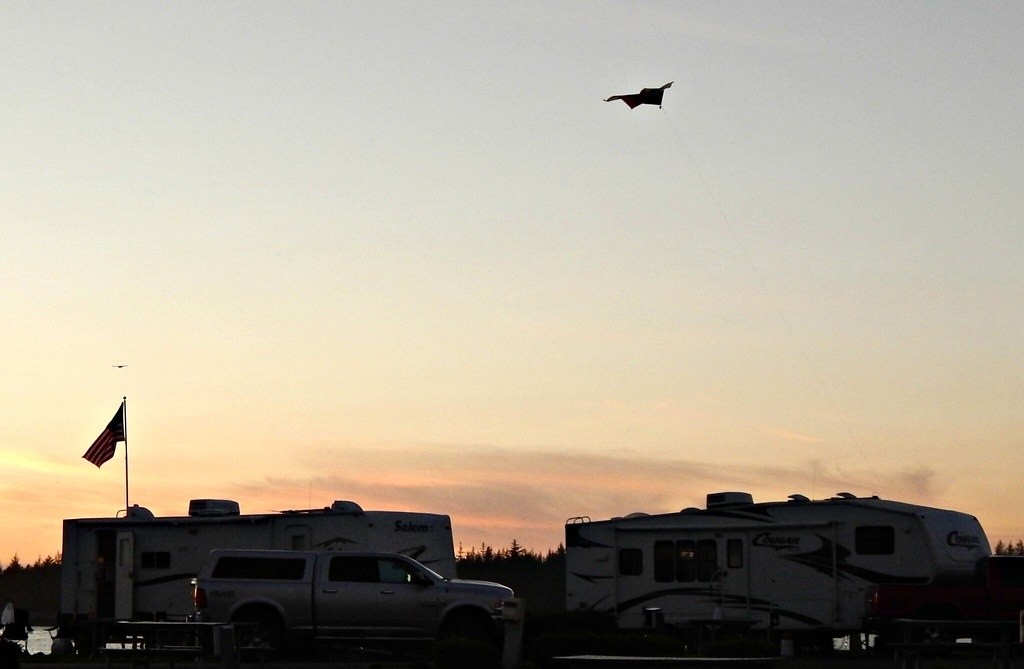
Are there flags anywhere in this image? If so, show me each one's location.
[82,402,124,468]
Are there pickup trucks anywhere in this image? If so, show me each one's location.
[187,549,515,669]
[862,555,1024,643]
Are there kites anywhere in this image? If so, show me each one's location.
[602,81,675,109]
